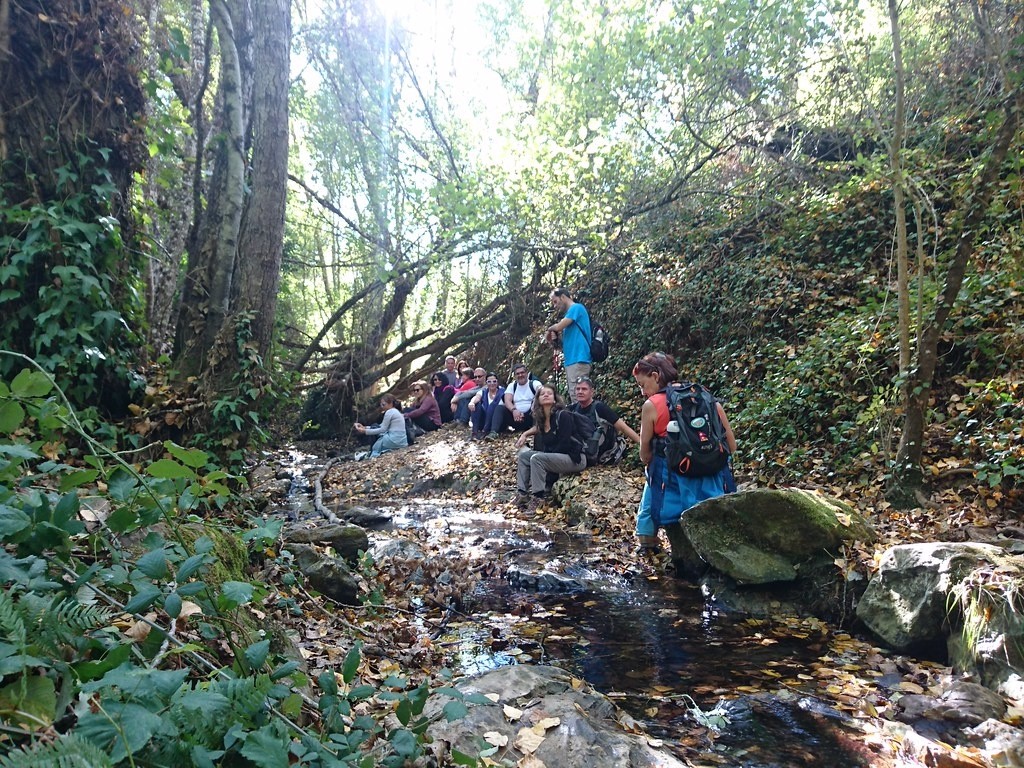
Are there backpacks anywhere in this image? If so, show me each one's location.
[595,408,627,465]
[555,409,605,461]
[648,381,731,479]
[514,373,544,395]
[572,307,612,362]
[405,417,425,445]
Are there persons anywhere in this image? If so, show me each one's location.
[546,287,594,403]
[484,363,545,442]
[515,377,640,496]
[402,379,442,435]
[467,372,506,441]
[431,355,477,423]
[446,367,487,431]
[632,351,739,579]
[353,393,408,460]
[509,383,589,517]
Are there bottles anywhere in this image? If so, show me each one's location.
[595,327,603,343]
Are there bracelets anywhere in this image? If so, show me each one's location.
[511,408,517,413]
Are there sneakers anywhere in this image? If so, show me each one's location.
[456,422,469,431]
[484,431,499,442]
[505,492,530,507]
[447,421,460,429]
[525,497,545,518]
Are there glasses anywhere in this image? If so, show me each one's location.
[433,378,443,381]
[459,366,467,368]
[412,389,423,392]
[639,351,665,376]
[474,374,486,379]
[487,379,498,384]
[379,402,387,406]
[514,371,527,375]
[575,377,591,383]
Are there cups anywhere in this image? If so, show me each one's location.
[593,426,604,440]
[667,421,680,441]
